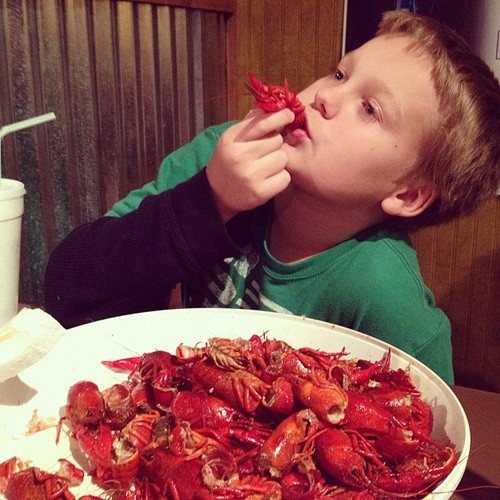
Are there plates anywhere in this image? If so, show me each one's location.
[0,307,471,500]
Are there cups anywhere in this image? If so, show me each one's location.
[0,177,27,324]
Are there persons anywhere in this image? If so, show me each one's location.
[45,10,500,387]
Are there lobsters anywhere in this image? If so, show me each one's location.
[190,72,306,134]
[0,335,500,500]
